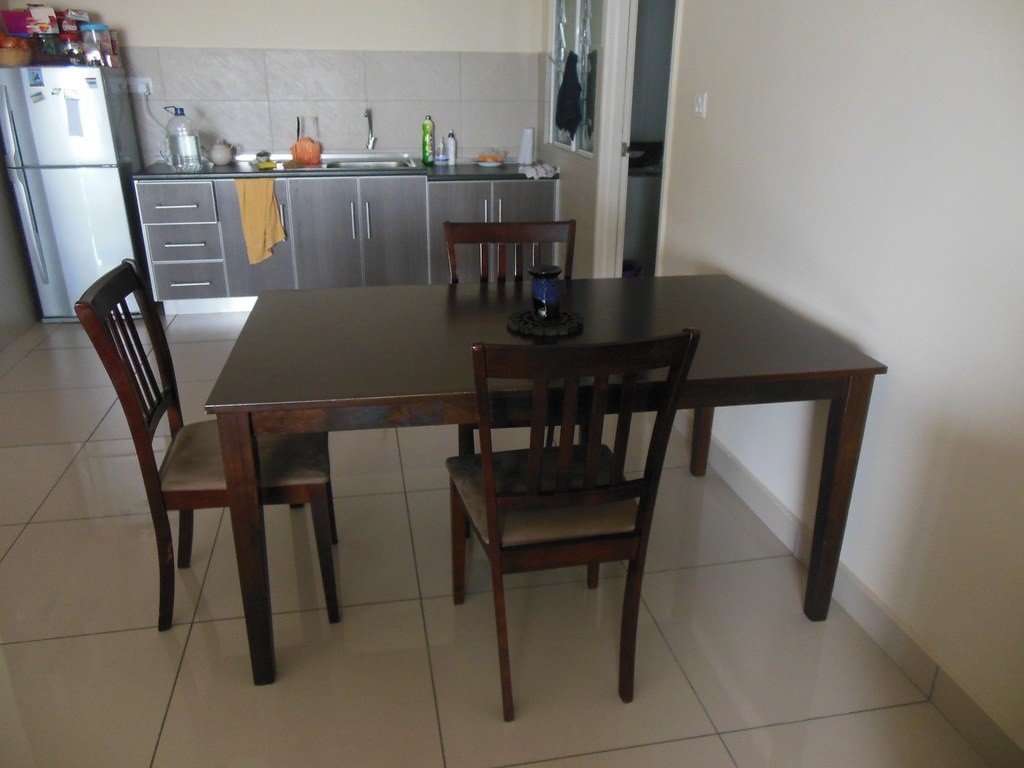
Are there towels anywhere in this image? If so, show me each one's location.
[234,177,285,264]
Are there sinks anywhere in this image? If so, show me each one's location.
[327,162,410,168]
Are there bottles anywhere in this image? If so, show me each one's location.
[446,130,456,166]
[60,23,120,67]
[164,106,202,173]
[528,265,562,322]
[422,115,436,165]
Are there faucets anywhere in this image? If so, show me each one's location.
[363,112,375,149]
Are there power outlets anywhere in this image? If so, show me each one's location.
[127,76,152,97]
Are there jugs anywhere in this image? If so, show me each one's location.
[200,138,237,165]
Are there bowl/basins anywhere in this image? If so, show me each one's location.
[0,48,33,66]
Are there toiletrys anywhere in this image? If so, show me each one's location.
[434,136,448,167]
[446,127,456,166]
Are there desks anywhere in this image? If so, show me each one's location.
[204,274,889,686]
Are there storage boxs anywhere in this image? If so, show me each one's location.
[0,3,89,36]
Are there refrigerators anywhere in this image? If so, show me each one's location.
[0,66,150,323]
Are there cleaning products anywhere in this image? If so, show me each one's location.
[422,112,435,166]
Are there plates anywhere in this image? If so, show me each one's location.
[478,161,503,167]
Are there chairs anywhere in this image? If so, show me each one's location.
[74,256,343,624]
[441,221,580,542]
[444,328,700,722]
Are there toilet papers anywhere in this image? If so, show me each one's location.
[517,127,534,164]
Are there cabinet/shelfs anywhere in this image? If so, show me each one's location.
[133,179,563,315]
